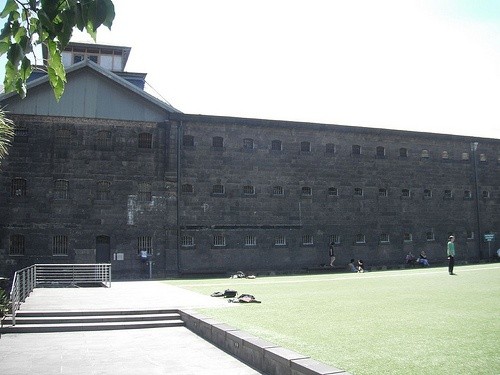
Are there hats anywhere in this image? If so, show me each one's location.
[331,242,335,245]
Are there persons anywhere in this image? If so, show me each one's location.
[329,242,364,274]
[447,236,456,275]
[406,249,430,268]
